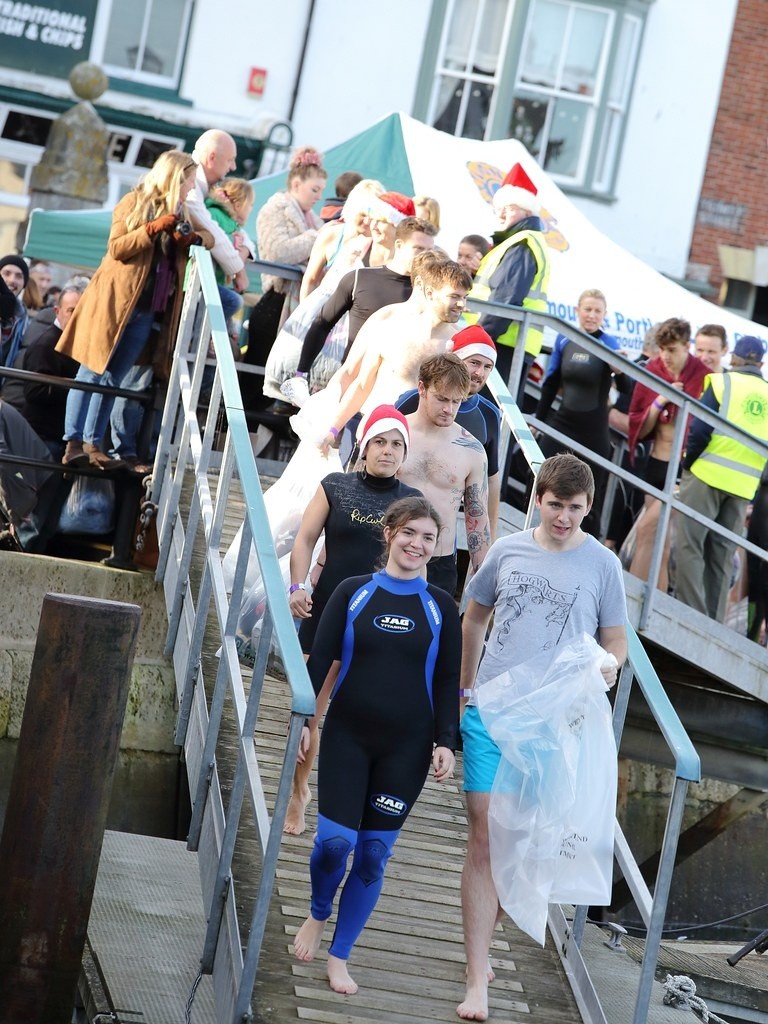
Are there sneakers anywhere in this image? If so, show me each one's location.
[82,443,126,470]
[61,441,89,465]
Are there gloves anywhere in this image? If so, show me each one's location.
[173,231,197,249]
[146,214,180,238]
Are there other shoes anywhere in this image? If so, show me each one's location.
[273,399,292,416]
[118,456,153,474]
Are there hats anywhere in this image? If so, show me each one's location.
[358,403,410,464]
[492,162,539,217]
[0,255,29,288]
[446,325,497,372]
[370,191,416,228]
[729,335,764,362]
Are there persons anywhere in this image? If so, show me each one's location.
[292,497,464,994]
[0,131,768,647]
[283,403,427,838]
[458,452,628,1022]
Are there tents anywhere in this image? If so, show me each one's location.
[23,114,767,370]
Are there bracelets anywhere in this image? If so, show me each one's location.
[289,584,306,595]
[459,688,472,697]
[653,399,662,411]
[316,561,323,567]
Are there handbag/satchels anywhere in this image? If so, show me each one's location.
[56,474,116,535]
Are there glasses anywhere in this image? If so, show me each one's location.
[183,158,199,171]
